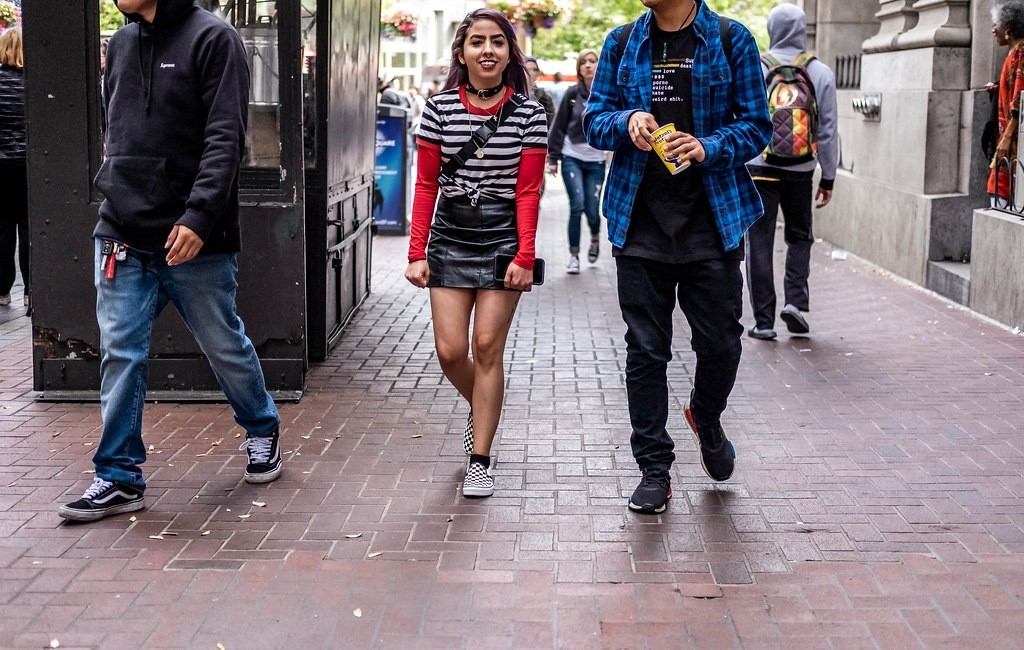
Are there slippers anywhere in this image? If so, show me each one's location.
[781,309,810,333]
[749,326,777,338]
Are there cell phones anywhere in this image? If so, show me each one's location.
[493,254,545,285]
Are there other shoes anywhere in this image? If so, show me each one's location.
[0,293,11,306]
[588,240,600,263]
[24,295,29,306]
[566,256,580,273]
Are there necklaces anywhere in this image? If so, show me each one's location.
[464,83,506,159]
[654,2,696,63]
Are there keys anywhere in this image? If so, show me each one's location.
[100,243,112,270]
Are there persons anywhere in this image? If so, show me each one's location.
[549,72,570,108]
[0,26,31,307]
[983,0,1023,207]
[521,56,556,130]
[404,8,547,497]
[582,0,774,514]
[741,0,838,337]
[60,0,282,519]
[548,49,607,273]
[375,73,440,151]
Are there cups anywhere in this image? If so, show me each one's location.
[645,123,691,176]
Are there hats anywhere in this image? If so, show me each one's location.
[377,77,385,89]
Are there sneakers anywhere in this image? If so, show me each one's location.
[463,461,494,496]
[683,397,735,481]
[628,477,672,513]
[238,427,283,483]
[58,475,144,520]
[462,405,475,456]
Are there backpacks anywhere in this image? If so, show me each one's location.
[761,49,818,167]
